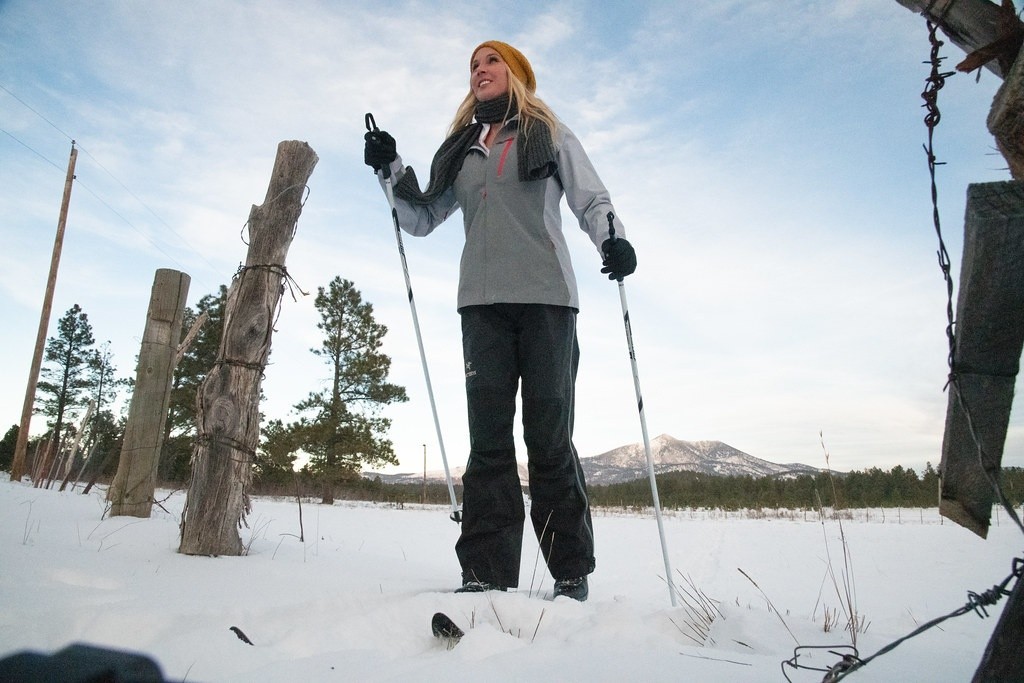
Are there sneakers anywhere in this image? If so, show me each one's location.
[554,573,589,602]
[455,578,517,593]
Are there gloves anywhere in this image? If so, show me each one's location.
[364,127,397,179]
[601,238,637,281]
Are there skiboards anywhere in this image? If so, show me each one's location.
[431,575,590,648]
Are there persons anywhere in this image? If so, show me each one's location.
[363,42,636,600]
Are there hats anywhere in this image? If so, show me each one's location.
[472,40,536,95]
